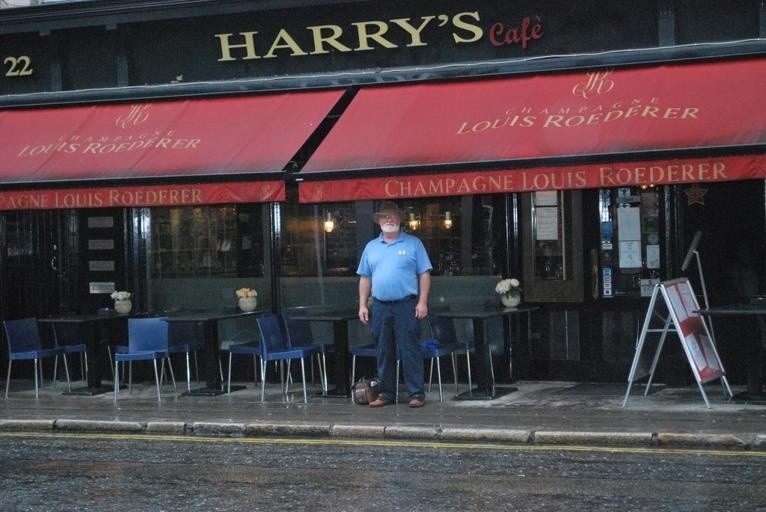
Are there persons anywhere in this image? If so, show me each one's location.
[237,240,261,277]
[358,201,434,408]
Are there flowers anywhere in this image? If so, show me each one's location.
[493,278,523,295]
[233,288,259,298]
[109,291,132,299]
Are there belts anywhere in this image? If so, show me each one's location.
[373,297,415,305]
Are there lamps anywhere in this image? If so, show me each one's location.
[439,209,453,229]
[323,211,335,232]
[403,207,422,232]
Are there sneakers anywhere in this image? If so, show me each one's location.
[408,398,425,406]
[369,398,389,406]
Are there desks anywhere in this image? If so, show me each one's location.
[34,313,152,394]
[692,302,765,403]
[163,310,266,393]
[435,307,540,401]
[287,309,372,398]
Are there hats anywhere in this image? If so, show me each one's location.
[372,201,407,225]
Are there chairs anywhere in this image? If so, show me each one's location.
[2,312,90,400]
[219,309,335,404]
[351,340,400,406]
[417,306,479,402]
[96,308,201,401]
[156,310,214,392]
